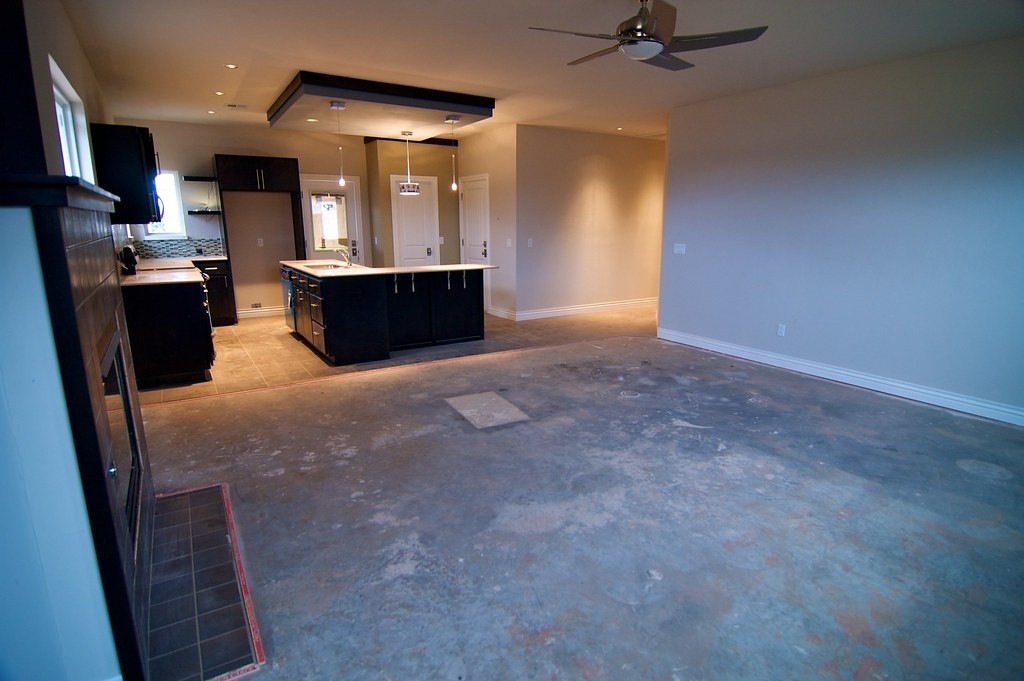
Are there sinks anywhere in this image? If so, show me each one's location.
[303,261,353,273]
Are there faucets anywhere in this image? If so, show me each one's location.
[333,247,355,266]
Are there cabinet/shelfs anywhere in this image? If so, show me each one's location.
[279,262,502,364]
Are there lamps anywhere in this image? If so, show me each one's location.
[329,101,348,188]
[445,114,462,191]
[397,129,422,196]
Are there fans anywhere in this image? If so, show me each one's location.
[528,0,768,73]
